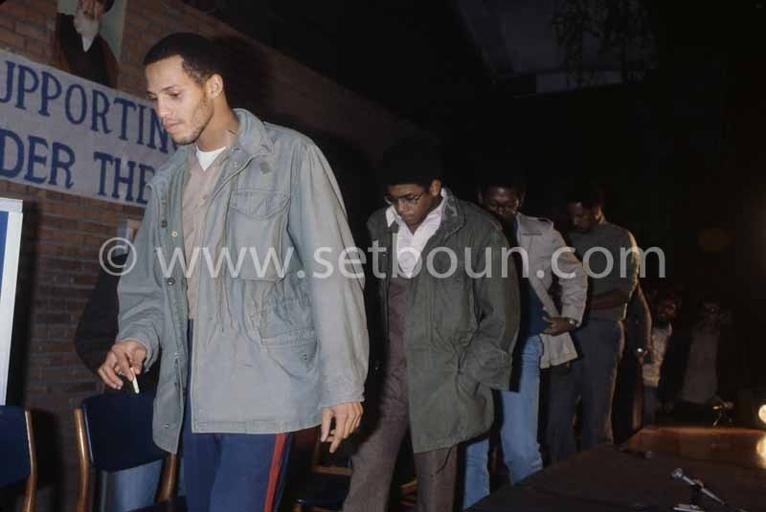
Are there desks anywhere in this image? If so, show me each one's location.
[618,416,766,470]
[455,445,766,512]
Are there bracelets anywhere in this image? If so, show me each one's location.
[567,318,576,325]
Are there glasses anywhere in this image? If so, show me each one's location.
[384,190,430,205]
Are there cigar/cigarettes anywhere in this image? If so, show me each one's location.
[130,368,140,394]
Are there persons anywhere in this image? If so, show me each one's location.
[537,173,640,466]
[612,286,735,443]
[340,161,521,512]
[73,253,164,512]
[56,0,117,88]
[97,32,370,512]
[462,171,588,512]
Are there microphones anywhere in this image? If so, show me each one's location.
[671,469,727,505]
[624,447,654,458]
[712,402,734,410]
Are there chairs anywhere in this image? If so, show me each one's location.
[290,425,507,512]
[73,392,177,511]
[0,403,38,511]
